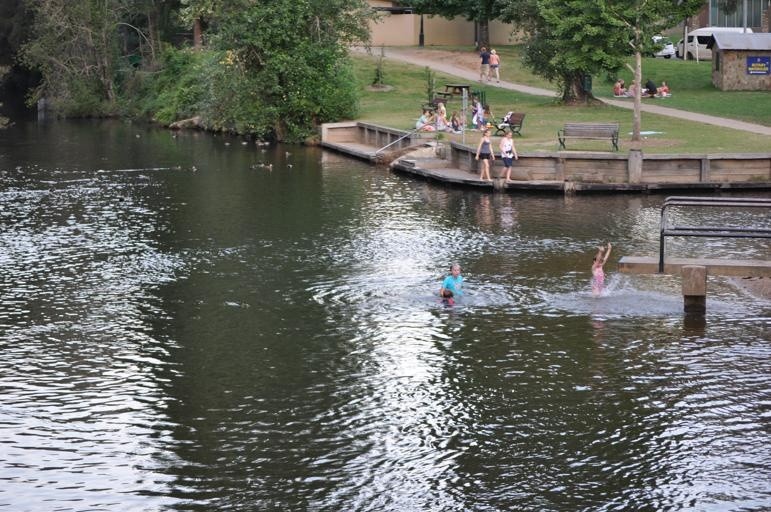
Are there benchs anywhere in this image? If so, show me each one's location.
[558,122,619,151]
[490,112,526,136]
[422,98,448,115]
[437,92,472,96]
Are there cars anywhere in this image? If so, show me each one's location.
[652,36,675,58]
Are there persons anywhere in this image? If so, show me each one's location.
[475,129,496,181]
[487,48,501,83]
[613,77,670,98]
[439,288,457,309]
[477,47,491,82]
[443,263,464,301]
[418,93,495,133]
[591,242,612,290]
[499,130,519,184]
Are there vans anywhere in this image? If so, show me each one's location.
[677,27,753,60]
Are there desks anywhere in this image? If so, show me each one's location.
[443,84,472,98]
